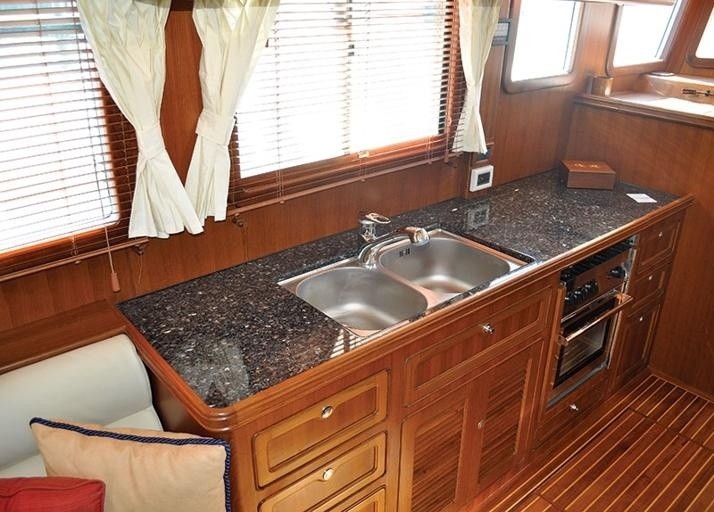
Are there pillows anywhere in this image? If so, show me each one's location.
[0,478,105,512]
[29,418,232,512]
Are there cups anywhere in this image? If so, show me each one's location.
[591,76,613,98]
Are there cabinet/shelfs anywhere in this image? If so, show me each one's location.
[601,208,687,400]
[523,369,612,475]
[402,270,553,512]
[145,263,401,511]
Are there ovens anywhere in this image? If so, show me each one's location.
[545,236,637,411]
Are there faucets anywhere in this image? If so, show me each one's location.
[356,207,431,267]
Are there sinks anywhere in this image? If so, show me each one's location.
[378,223,536,294]
[275,257,428,338]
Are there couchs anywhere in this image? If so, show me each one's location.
[0,334,162,478]
